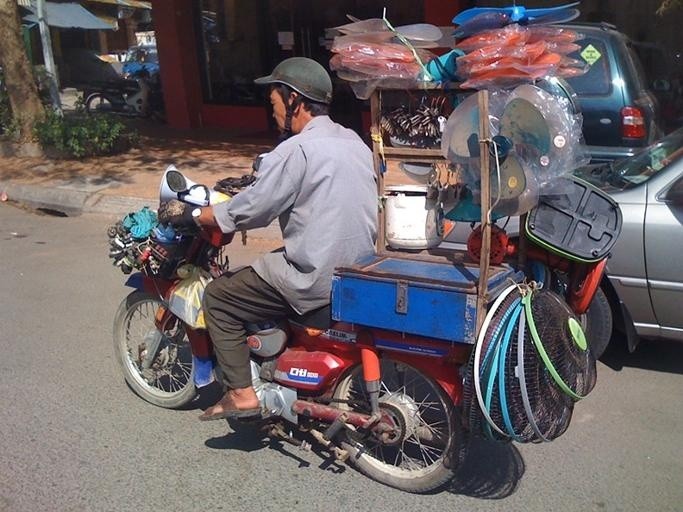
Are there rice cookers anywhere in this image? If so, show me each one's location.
[383,183,444,251]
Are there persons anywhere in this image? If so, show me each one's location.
[157,58,377,420]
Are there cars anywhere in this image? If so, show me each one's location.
[435,130,683,361]
[115,43,160,80]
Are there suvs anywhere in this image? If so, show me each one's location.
[508,22,672,167]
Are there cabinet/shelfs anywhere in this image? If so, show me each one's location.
[378,84,488,269]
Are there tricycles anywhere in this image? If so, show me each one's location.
[106,167,529,497]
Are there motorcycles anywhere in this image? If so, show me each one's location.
[80,65,157,121]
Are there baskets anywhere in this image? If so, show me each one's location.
[141,237,195,279]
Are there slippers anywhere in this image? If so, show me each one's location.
[198,390,262,421]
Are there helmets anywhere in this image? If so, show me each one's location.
[253,57,333,106]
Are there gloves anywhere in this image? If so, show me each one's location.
[159,198,202,229]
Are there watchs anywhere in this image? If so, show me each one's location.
[192,206,205,230]
